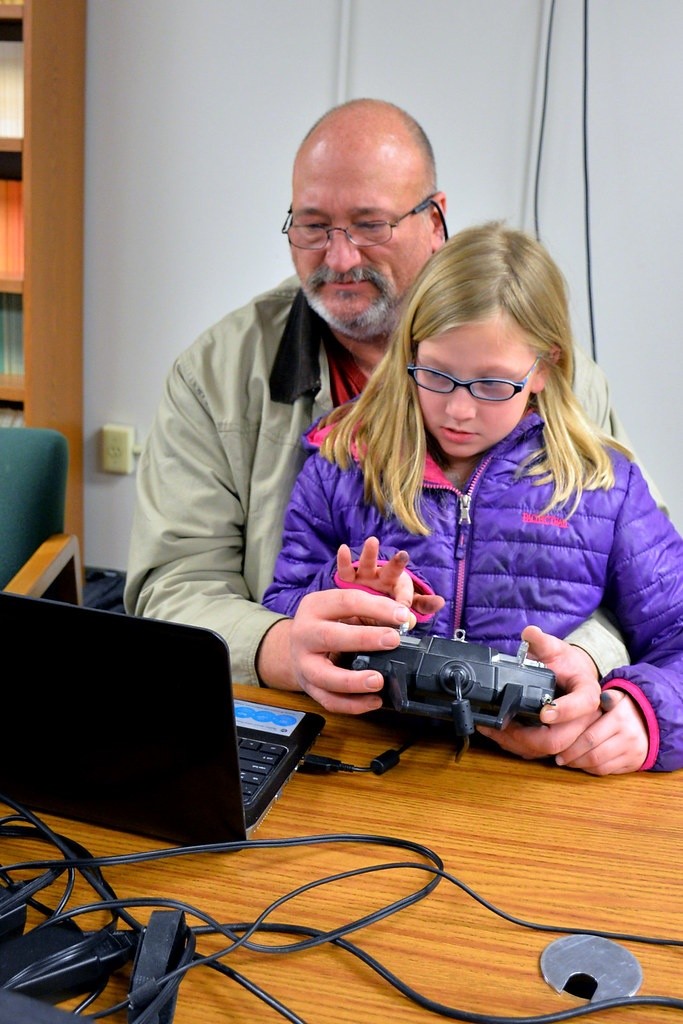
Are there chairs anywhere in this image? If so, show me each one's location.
[0,424,84,608]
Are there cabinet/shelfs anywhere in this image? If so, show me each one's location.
[0,0,91,560]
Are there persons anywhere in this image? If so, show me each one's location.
[123,99,632,760]
[262,223,683,775]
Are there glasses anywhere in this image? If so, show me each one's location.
[282,197,432,249]
[407,341,542,402]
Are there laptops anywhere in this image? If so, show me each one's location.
[0,590,325,853]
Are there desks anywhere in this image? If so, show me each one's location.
[0,680,683,1023]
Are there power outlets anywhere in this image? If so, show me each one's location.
[100,425,137,473]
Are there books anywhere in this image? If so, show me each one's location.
[0,41,25,427]
